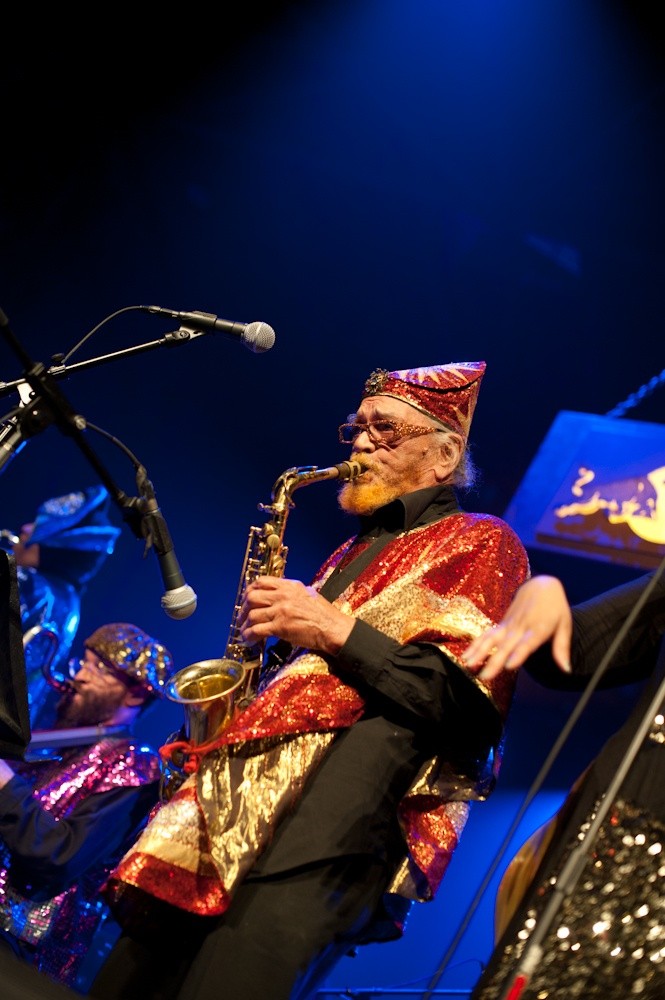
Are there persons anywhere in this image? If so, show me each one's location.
[1,621,176,993]
[0,485,123,734]
[90,357,534,1000]
[458,559,665,1000]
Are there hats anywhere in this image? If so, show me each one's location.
[85,622,173,697]
[363,361,487,438]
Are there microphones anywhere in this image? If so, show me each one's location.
[140,304,277,354]
[136,466,197,621]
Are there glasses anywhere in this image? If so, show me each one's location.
[79,659,132,685]
[338,419,449,451]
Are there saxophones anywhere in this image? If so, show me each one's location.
[162,457,364,750]
